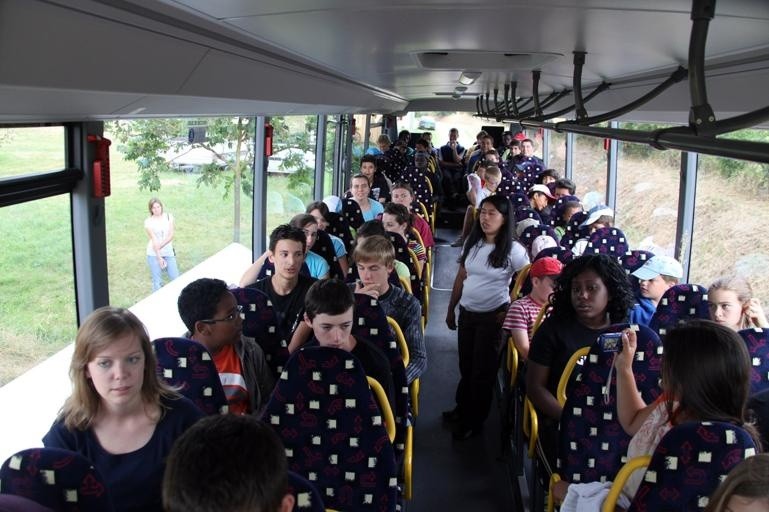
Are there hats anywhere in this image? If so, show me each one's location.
[513,133,528,141]
[515,218,541,238]
[530,235,558,263]
[530,184,556,200]
[530,256,566,278]
[322,196,343,214]
[578,205,614,231]
[629,255,684,281]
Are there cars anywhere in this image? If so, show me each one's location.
[419,116,435,131]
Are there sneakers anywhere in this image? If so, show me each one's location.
[449,236,464,247]
[451,191,460,200]
[440,410,478,453]
[455,254,465,264]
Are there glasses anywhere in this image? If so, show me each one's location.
[198,305,244,324]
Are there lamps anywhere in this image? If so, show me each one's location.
[459,71,482,86]
[452,95,461,101]
[454,87,468,96]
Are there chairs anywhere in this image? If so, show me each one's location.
[463,126,768,510]
[2,130,436,511]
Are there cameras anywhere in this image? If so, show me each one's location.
[597,332,629,353]
